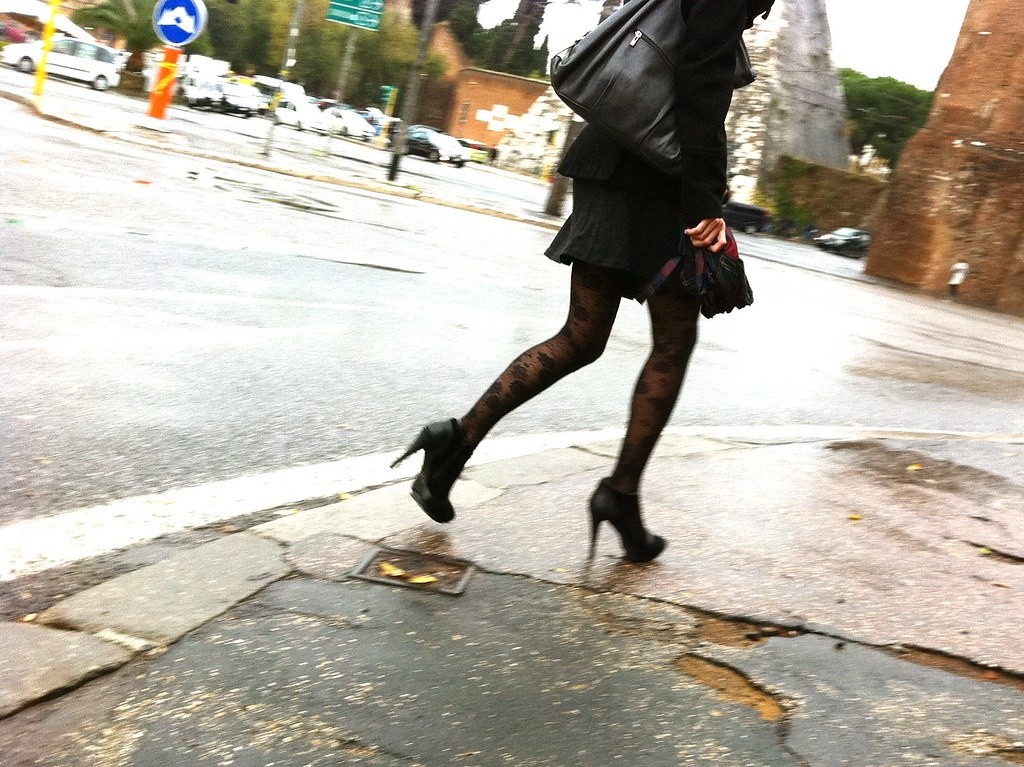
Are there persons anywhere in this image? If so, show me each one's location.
[946,255,970,301]
[384,2,784,562]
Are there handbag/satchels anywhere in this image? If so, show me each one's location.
[549,0,758,175]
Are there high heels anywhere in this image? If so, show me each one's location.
[590,478,664,563]
[391,419,461,523]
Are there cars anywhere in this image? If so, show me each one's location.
[816,225,872,259]
[723,197,771,235]
[0,13,500,170]
